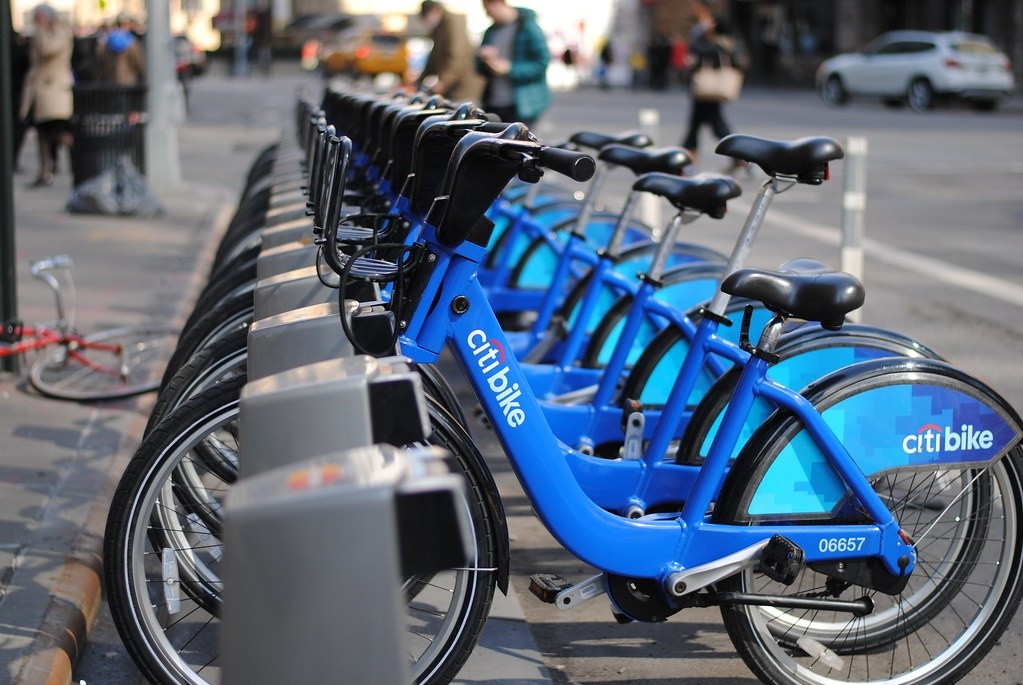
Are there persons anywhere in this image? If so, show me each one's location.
[16,4,75,187]
[474,0,554,136]
[558,1,820,98]
[78,11,144,91]
[400,0,486,110]
[673,10,753,179]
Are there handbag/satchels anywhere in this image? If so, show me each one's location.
[689,49,742,100]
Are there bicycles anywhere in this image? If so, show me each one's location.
[99,75,1023,685]
[0,251,184,405]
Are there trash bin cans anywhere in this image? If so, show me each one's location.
[70,80,147,188]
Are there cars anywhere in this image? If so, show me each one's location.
[815,30,1016,114]
[317,30,409,81]
[171,10,385,76]
